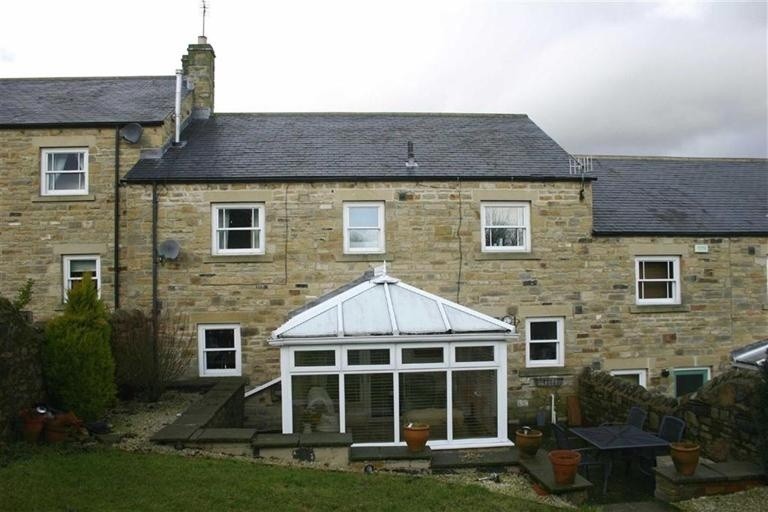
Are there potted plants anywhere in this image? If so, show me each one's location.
[303,409,321,432]
[516,425,543,455]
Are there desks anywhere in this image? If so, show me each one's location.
[567,424,671,494]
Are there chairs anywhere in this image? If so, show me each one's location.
[659,415,686,441]
[627,407,648,429]
[550,422,610,495]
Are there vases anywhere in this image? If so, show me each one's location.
[17,407,68,443]
[548,450,582,484]
[670,442,699,476]
[403,422,431,452]
